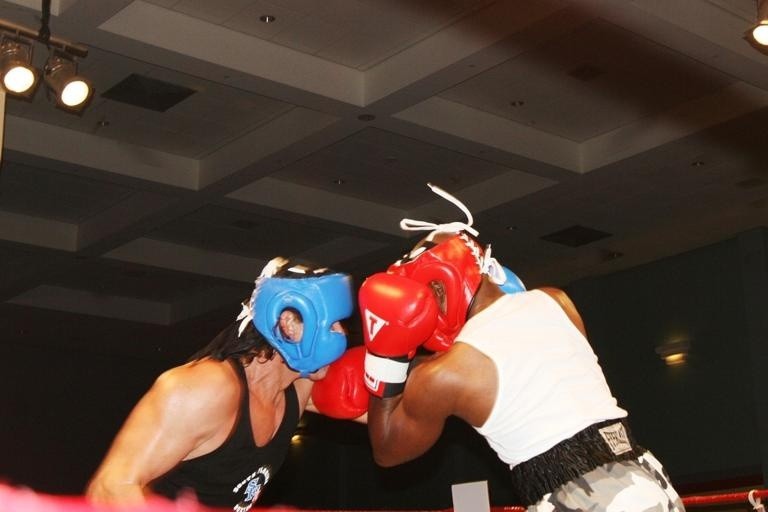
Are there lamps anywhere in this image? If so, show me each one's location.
[0,30,93,115]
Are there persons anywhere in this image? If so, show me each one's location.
[86,253,529,512]
[310,184,686,512]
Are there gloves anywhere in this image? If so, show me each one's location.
[498,267,527,295]
[358,273,440,399]
[310,344,366,420]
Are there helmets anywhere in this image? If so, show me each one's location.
[388,232,486,355]
[250,272,355,377]
